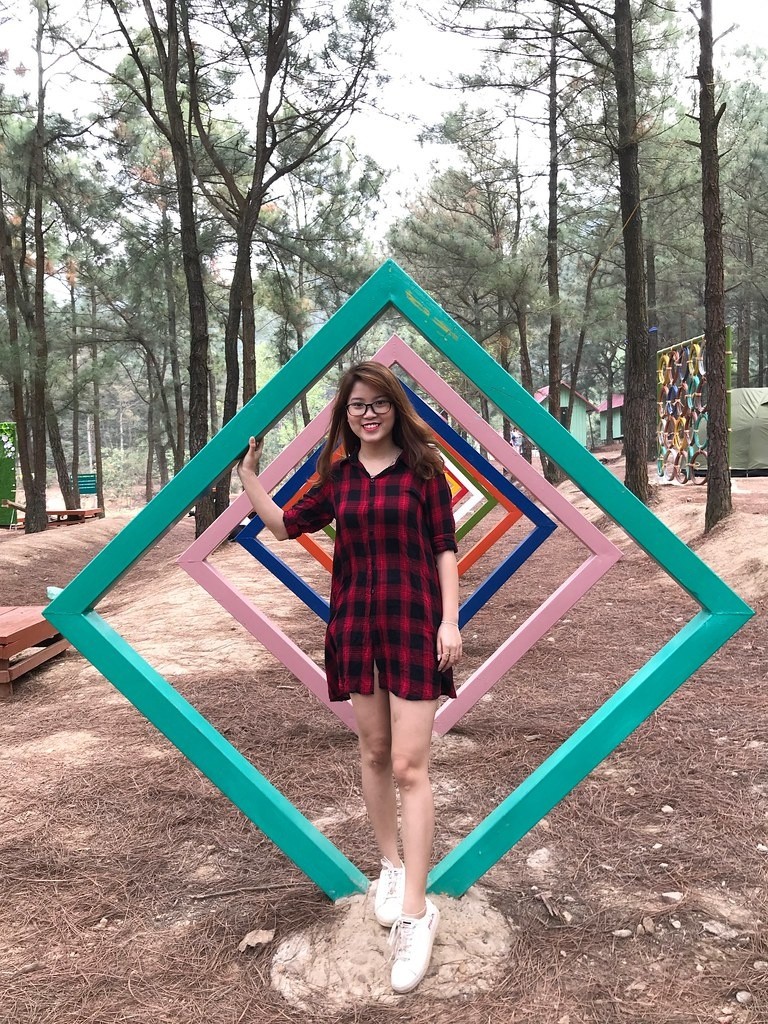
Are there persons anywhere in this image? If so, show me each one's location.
[233,354,471,994]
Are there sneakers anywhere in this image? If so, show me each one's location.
[389,898,440,993]
[374,858,406,928]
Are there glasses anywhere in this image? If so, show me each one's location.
[344,399,393,417]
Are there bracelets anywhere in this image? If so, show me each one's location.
[440,619,461,628]
